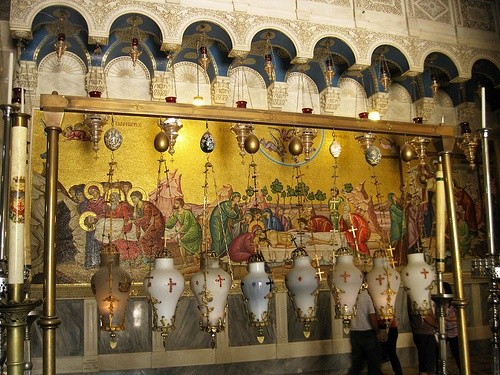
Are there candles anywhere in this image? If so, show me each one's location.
[437,153,443,163]
[21,85,25,114]
[441,112,448,124]
[7,51,14,106]
[481,85,488,129]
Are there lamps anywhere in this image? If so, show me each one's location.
[368,104,382,122]
[52,13,67,63]
[403,163,435,326]
[128,18,143,68]
[192,64,204,107]
[189,160,235,350]
[90,163,132,350]
[365,166,401,334]
[142,159,186,348]
[284,164,325,340]
[239,163,276,345]
[329,161,364,335]
